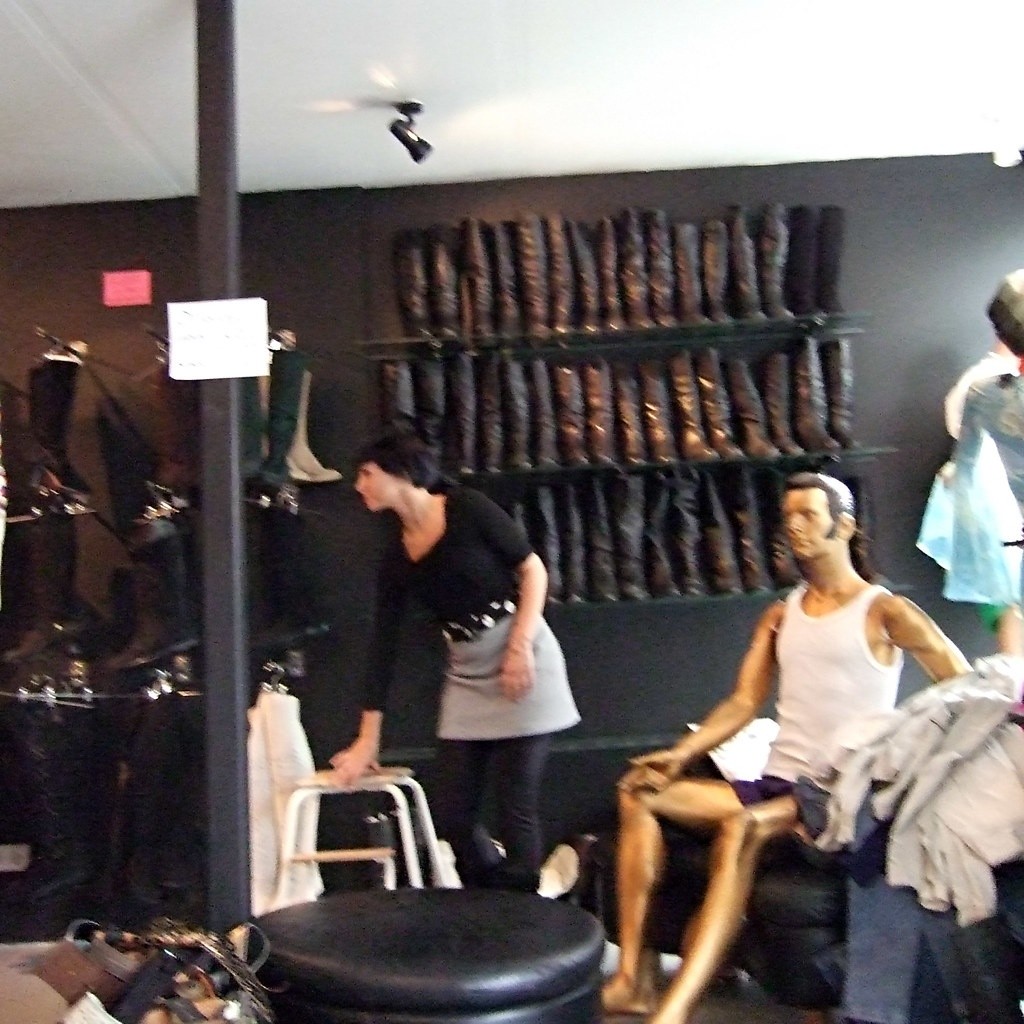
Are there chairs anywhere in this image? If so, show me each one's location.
[259,694,449,888]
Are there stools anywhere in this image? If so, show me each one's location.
[758,869,843,1005]
[234,888,607,1024]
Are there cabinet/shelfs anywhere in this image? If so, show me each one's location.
[355,313,916,618]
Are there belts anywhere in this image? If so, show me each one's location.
[438,598,520,645]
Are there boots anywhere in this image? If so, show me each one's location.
[0,342,342,694]
[378,201,877,605]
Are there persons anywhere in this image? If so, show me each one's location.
[330,421,574,897]
[602,472,975,1024]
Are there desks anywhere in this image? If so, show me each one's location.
[0,654,207,936]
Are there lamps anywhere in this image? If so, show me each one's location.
[391,101,435,164]
